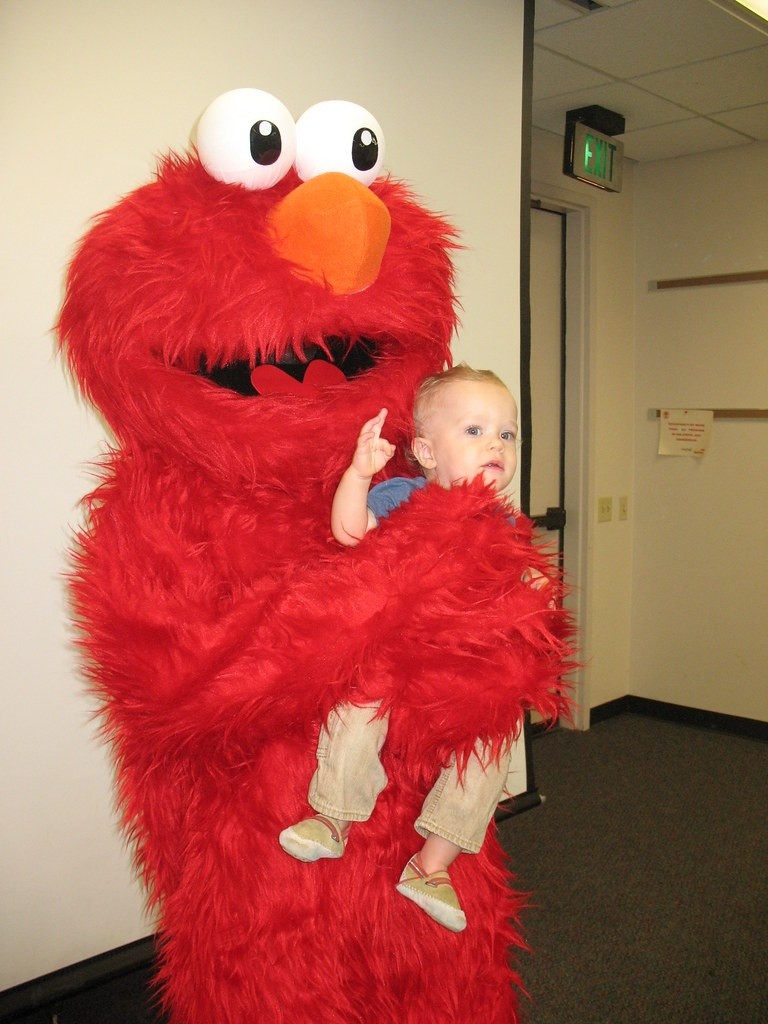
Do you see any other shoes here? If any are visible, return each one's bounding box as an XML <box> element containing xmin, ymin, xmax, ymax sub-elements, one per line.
<box><xmin>278</xmin><ymin>814</ymin><xmax>353</xmax><ymax>863</ymax></box>
<box><xmin>394</xmin><ymin>852</ymin><xmax>467</xmax><ymax>932</ymax></box>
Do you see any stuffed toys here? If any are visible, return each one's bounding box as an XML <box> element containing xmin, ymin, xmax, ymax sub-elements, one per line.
<box><xmin>62</xmin><ymin>86</ymin><xmax>563</xmax><ymax>1024</ymax></box>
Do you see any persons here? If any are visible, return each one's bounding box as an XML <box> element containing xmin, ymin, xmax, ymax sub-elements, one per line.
<box><xmin>281</xmin><ymin>364</ymin><xmax>550</xmax><ymax>931</ymax></box>
